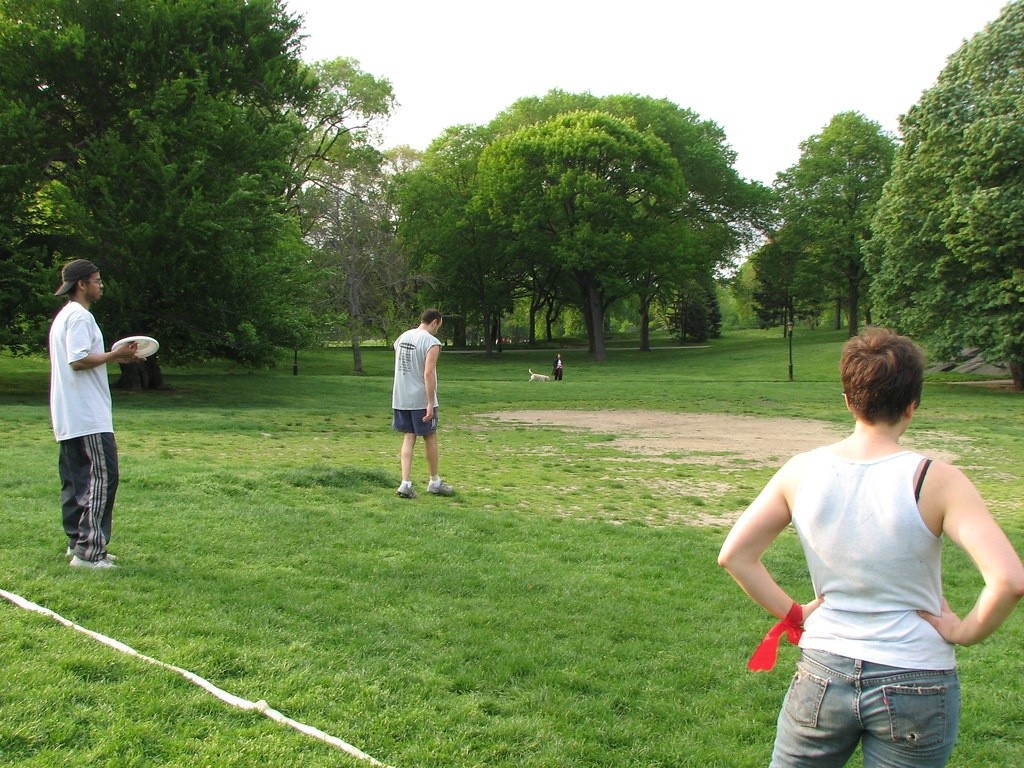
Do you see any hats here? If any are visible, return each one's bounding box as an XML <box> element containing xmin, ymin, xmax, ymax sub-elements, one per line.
<box><xmin>54</xmin><ymin>259</ymin><xmax>99</xmax><ymax>297</ymax></box>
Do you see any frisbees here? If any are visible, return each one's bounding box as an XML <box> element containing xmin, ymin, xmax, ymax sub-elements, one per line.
<box><xmin>110</xmin><ymin>335</ymin><xmax>161</xmax><ymax>359</ymax></box>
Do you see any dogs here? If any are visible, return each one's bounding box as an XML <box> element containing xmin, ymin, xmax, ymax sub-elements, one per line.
<box><xmin>528</xmin><ymin>369</ymin><xmax>550</xmax><ymax>382</ymax></box>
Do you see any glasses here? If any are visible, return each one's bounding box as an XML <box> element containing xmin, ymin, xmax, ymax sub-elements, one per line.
<box><xmin>85</xmin><ymin>277</ymin><xmax>103</xmax><ymax>284</ymax></box>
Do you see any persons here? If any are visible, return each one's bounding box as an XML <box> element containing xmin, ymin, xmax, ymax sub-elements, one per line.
<box><xmin>718</xmin><ymin>328</ymin><xmax>1024</xmax><ymax>768</ymax></box>
<box><xmin>552</xmin><ymin>354</ymin><xmax>562</xmax><ymax>381</ymax></box>
<box><xmin>392</xmin><ymin>309</ymin><xmax>453</xmax><ymax>498</ymax></box>
<box><xmin>48</xmin><ymin>259</ymin><xmax>146</xmax><ymax>569</ymax></box>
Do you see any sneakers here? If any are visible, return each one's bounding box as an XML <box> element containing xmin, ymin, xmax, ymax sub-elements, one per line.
<box><xmin>427</xmin><ymin>480</ymin><xmax>454</xmax><ymax>495</ymax></box>
<box><xmin>395</xmin><ymin>484</ymin><xmax>417</xmax><ymax>498</ymax></box>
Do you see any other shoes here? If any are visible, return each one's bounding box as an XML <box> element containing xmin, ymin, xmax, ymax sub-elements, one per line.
<box><xmin>66</xmin><ymin>546</ymin><xmax>119</xmax><ymax>560</ymax></box>
<box><xmin>70</xmin><ymin>555</ymin><xmax>118</xmax><ymax>569</ymax></box>
